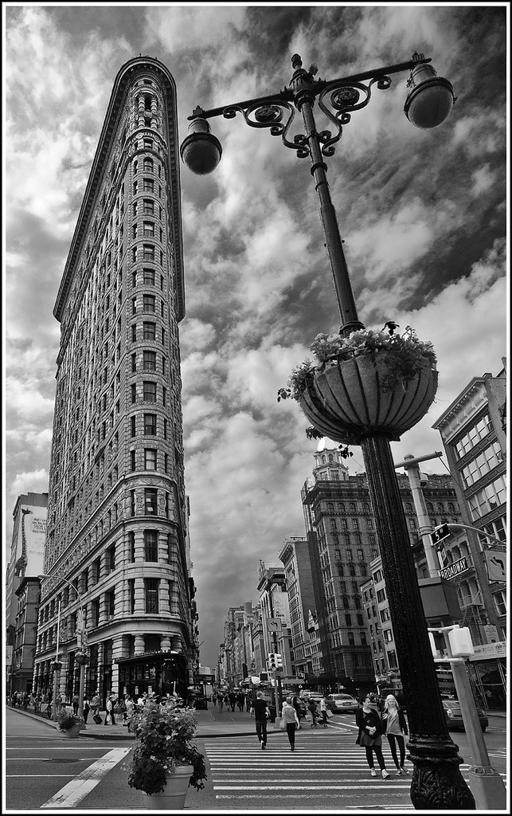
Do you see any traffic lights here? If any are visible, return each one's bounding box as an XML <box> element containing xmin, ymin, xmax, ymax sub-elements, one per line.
<box><xmin>276</xmin><ymin>654</ymin><xmax>282</xmax><ymax>669</ymax></box>
<box><xmin>268</xmin><ymin>653</ymin><xmax>278</xmax><ymax>670</ymax></box>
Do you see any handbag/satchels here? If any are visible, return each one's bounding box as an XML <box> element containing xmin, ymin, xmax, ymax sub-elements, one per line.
<box><xmin>325</xmin><ymin>709</ymin><xmax>334</xmax><ymax>718</ymax></box>
<box><xmin>85</xmin><ymin>703</ymin><xmax>89</xmax><ymax>709</ymax></box>
<box><xmin>362</xmin><ymin>732</ymin><xmax>377</xmax><ymax>747</ymax></box>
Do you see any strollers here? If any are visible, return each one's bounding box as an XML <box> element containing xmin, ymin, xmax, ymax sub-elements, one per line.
<box><xmin>310</xmin><ymin>709</ymin><xmax>328</xmax><ymax>729</ymax></box>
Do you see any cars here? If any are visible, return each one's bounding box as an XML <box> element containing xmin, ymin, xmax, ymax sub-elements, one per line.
<box><xmin>213</xmin><ymin>685</ymin><xmax>359</xmax><ymax>719</ymax></box>
<box><xmin>439</xmin><ymin>694</ymin><xmax>488</xmax><ymax>734</ymax></box>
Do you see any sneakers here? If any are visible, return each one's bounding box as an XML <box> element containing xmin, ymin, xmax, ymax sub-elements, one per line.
<box><xmin>380</xmin><ymin>768</ymin><xmax>392</xmax><ymax>779</ymax></box>
<box><xmin>395</xmin><ymin>770</ymin><xmax>403</xmax><ymax>778</ymax></box>
<box><xmin>260</xmin><ymin>740</ymin><xmax>265</xmax><ymax>749</ymax></box>
<box><xmin>402</xmin><ymin>765</ymin><xmax>411</xmax><ymax>777</ymax></box>
<box><xmin>368</xmin><ymin>768</ymin><xmax>377</xmax><ymax>778</ymax></box>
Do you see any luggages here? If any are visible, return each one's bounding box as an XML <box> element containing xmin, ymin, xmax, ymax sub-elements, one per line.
<box><xmin>88</xmin><ymin>709</ymin><xmax>103</xmax><ymax>725</ymax></box>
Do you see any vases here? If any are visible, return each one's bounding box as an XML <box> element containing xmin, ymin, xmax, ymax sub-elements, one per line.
<box><xmin>298</xmin><ymin>355</ymin><xmax>437</xmax><ymax>443</ymax></box>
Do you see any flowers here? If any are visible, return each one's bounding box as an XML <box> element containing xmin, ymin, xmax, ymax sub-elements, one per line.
<box><xmin>276</xmin><ymin>318</ymin><xmax>437</xmax><ymax>403</ymax></box>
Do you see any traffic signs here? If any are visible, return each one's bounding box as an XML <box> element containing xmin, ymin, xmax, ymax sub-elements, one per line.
<box><xmin>437</xmin><ymin>555</ymin><xmax>470</xmax><ymax>582</ymax></box>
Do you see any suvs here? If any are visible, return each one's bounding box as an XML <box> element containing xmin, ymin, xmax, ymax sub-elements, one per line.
<box><xmin>376</xmin><ymin>688</ymin><xmax>408</xmax><ymax>714</ymax></box>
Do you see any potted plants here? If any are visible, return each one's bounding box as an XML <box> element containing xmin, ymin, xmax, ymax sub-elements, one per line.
<box><xmin>123</xmin><ymin>690</ymin><xmax>208</xmax><ymax>811</ymax></box>
<box><xmin>56</xmin><ymin>710</ymin><xmax>85</xmax><ymax>739</ymax></box>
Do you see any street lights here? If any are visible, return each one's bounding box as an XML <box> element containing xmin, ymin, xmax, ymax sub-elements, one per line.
<box><xmin>257</xmin><ymin>573</ymin><xmax>285</xmax><ymax>717</ymax></box>
<box><xmin>38</xmin><ymin>575</ymin><xmax>87</xmax><ymax>730</ymax></box>
<box><xmin>179</xmin><ymin>53</ymin><xmax>476</xmax><ymax>810</ymax></box>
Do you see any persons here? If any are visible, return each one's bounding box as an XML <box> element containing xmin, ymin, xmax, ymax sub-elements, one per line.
<box><xmin>10</xmin><ymin>690</ymin><xmax>147</xmax><ymax>726</ymax></box>
<box><xmin>211</xmin><ymin>688</ymin><xmax>328</xmax><ymax>751</ymax></box>
<box><xmin>381</xmin><ymin>695</ymin><xmax>410</xmax><ymax>776</ymax></box>
<box><xmin>355</xmin><ymin>697</ymin><xmax>391</xmax><ymax>779</ymax></box>
